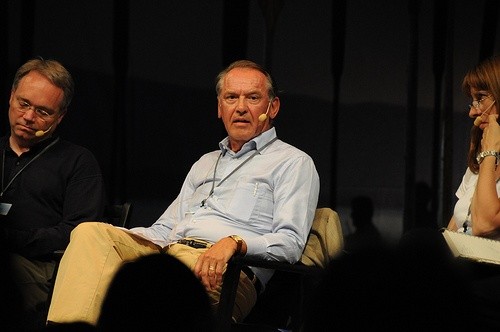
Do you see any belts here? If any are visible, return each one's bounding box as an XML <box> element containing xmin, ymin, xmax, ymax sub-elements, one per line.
<box><xmin>178</xmin><ymin>240</ymin><xmax>263</xmax><ymax>302</ymax></box>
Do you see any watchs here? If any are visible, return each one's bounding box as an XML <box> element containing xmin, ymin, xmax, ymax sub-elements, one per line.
<box><xmin>228</xmin><ymin>234</ymin><xmax>242</xmax><ymax>255</ymax></box>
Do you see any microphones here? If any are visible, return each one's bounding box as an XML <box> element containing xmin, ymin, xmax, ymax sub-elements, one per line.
<box><xmin>35</xmin><ymin>120</ymin><xmax>58</xmax><ymax>137</ymax></box>
<box><xmin>473</xmin><ymin>100</ymin><xmax>496</xmax><ymax>127</ymax></box>
<box><xmin>258</xmin><ymin>98</ymin><xmax>273</xmax><ymax>121</ymax></box>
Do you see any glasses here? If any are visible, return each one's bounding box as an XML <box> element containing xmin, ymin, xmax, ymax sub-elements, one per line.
<box><xmin>468</xmin><ymin>93</ymin><xmax>493</xmax><ymax>110</ymax></box>
<box><xmin>13</xmin><ymin>90</ymin><xmax>60</xmax><ymax>121</ymax></box>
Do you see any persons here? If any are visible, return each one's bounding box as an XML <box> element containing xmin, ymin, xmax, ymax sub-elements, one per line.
<box><xmin>95</xmin><ymin>253</ymin><xmax>214</xmax><ymax>332</ymax></box>
<box><xmin>0</xmin><ymin>57</ymin><xmax>104</xmax><ymax>326</ymax></box>
<box><xmin>385</xmin><ymin>52</ymin><xmax>500</xmax><ymax>331</ymax></box>
<box><xmin>46</xmin><ymin>60</ymin><xmax>322</xmax><ymax>332</ymax></box>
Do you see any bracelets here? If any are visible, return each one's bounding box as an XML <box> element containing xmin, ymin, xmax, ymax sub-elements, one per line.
<box><xmin>476</xmin><ymin>150</ymin><xmax>498</xmax><ymax>165</ymax></box>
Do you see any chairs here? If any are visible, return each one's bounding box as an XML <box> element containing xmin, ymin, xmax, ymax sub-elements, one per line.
<box><xmin>216</xmin><ymin>208</ymin><xmax>343</xmax><ymax>332</ymax></box>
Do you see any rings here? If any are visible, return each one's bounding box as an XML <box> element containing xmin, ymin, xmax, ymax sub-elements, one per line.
<box><xmin>209</xmin><ymin>267</ymin><xmax>215</xmax><ymax>271</ymax></box>
